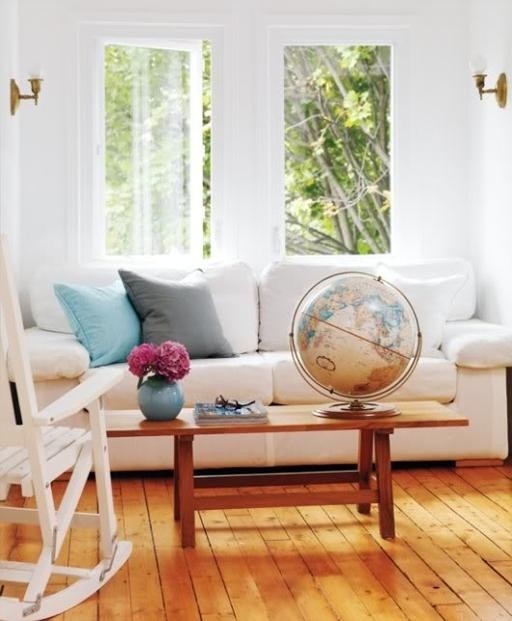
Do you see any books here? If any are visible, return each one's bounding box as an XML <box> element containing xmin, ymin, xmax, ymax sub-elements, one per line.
<box><xmin>193</xmin><ymin>402</ymin><xmax>270</xmax><ymax>425</ymax></box>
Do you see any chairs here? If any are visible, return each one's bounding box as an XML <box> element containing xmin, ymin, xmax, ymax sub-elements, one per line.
<box><xmin>1</xmin><ymin>233</ymin><xmax>138</xmax><ymax>621</ymax></box>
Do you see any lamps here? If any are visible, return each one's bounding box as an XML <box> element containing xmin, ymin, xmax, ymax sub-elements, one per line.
<box><xmin>11</xmin><ymin>51</ymin><xmax>43</xmax><ymax>113</ymax></box>
<box><xmin>468</xmin><ymin>51</ymin><xmax>508</xmax><ymax>107</ymax></box>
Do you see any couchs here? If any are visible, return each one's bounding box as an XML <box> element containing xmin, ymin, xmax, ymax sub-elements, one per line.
<box><xmin>14</xmin><ymin>251</ymin><xmax>510</xmax><ymax>475</ymax></box>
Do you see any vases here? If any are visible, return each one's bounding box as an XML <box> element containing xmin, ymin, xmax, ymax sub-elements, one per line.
<box><xmin>137</xmin><ymin>378</ymin><xmax>185</xmax><ymax>421</ymax></box>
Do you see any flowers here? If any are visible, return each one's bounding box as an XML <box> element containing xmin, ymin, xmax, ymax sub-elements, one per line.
<box><xmin>127</xmin><ymin>341</ymin><xmax>192</xmax><ymax>387</ymax></box>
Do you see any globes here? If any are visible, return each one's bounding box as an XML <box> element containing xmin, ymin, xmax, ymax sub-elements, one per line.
<box><xmin>288</xmin><ymin>271</ymin><xmax>422</xmax><ymax>420</ymax></box>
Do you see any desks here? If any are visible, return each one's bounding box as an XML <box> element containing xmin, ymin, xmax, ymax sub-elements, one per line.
<box><xmin>98</xmin><ymin>399</ymin><xmax>470</xmax><ymax>548</ymax></box>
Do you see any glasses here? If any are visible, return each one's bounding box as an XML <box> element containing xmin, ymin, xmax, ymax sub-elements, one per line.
<box><xmin>215</xmin><ymin>394</ymin><xmax>255</xmax><ymax>410</ymax></box>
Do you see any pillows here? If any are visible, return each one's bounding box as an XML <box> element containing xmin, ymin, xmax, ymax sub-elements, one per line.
<box><xmin>51</xmin><ymin>277</ymin><xmax>141</xmax><ymax>368</ymax></box>
<box><xmin>373</xmin><ymin>264</ymin><xmax>457</xmax><ymax>360</ymax></box>
<box><xmin>117</xmin><ymin>263</ymin><xmax>236</xmax><ymax>359</ymax></box>
